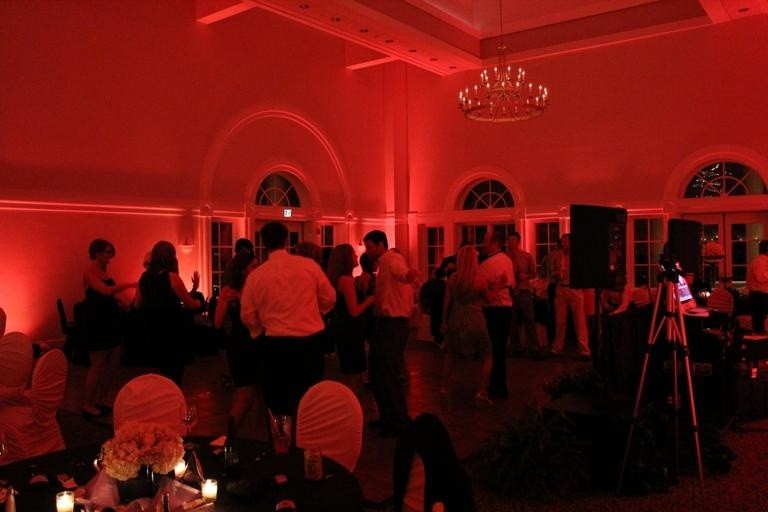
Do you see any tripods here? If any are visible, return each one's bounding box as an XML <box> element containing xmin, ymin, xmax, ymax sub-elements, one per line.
<box><xmin>627</xmin><ymin>273</ymin><xmax>709</xmax><ymax>492</ymax></box>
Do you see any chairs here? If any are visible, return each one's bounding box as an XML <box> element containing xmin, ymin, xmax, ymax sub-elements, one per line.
<box><xmin>2</xmin><ymin>331</ymin><xmax>33</xmax><ymax>406</ymax></box>
<box><xmin>1</xmin><ymin>348</ymin><xmax>67</xmax><ymax>464</ymax></box>
<box><xmin>297</xmin><ymin>380</ymin><xmax>362</xmax><ymax>471</ymax></box>
<box><xmin>113</xmin><ymin>375</ymin><xmax>188</xmax><ymax>436</ymax></box>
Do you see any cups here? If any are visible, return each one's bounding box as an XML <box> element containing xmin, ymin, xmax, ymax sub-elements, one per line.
<box><xmin>304</xmin><ymin>449</ymin><xmax>322</xmax><ymax>480</ymax></box>
<box><xmin>175</xmin><ymin>458</ymin><xmax>184</xmax><ymax>476</ymax></box>
<box><xmin>201</xmin><ymin>478</ymin><xmax>217</xmax><ymax>500</ymax></box>
<box><xmin>272</xmin><ymin>415</ymin><xmax>292</xmax><ymax>455</ymax></box>
<box><xmin>56</xmin><ymin>490</ymin><xmax>75</xmax><ymax>512</ymax></box>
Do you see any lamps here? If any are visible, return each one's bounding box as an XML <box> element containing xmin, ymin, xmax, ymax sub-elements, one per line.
<box><xmin>458</xmin><ymin>0</ymin><xmax>550</xmax><ymax>124</ymax></box>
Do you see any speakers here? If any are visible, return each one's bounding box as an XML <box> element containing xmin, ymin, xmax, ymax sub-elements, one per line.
<box><xmin>569</xmin><ymin>204</ymin><xmax>627</xmax><ymax>291</ymax></box>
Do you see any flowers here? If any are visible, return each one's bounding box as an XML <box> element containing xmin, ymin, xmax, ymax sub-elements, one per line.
<box><xmin>101</xmin><ymin>423</ymin><xmax>184</xmax><ymax>480</ymax></box>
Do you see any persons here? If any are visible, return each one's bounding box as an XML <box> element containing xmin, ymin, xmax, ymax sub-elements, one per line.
<box><xmin>241</xmin><ymin>222</ymin><xmax>336</xmax><ymax>443</ymax></box>
<box><xmin>357</xmin><ymin>252</ymin><xmax>379</xmax><ymax>388</ymax></box>
<box><xmin>216</xmin><ymin>252</ymin><xmax>257</xmax><ymax>440</ymax></box>
<box><xmin>430</xmin><ymin>240</ymin><xmax>507</xmax><ymax>406</ymax></box>
<box><xmin>479</xmin><ymin>230</ymin><xmax>516</xmax><ymax>400</ymax></box>
<box><xmin>539</xmin><ymin>235</ymin><xmax>590</xmax><ymax>356</ymax></box>
<box><xmin>71</xmin><ymin>237</ymin><xmax>138</xmax><ymax>417</ymax></box>
<box><xmin>598</xmin><ymin>239</ymin><xmax>768</xmax><ymax>339</ymax></box>
<box><xmin>326</xmin><ymin>243</ymin><xmax>377</xmax><ymax>392</ymax></box>
<box><xmin>505</xmin><ymin>231</ymin><xmax>546</xmax><ymax>360</ymax></box>
<box><xmin>235</xmin><ymin>238</ymin><xmax>261</xmax><ymax>268</ymax></box>
<box><xmin>132</xmin><ymin>241</ymin><xmax>205</xmax><ymax>390</ymax></box>
<box><xmin>364</xmin><ymin>229</ymin><xmax>420</xmax><ymax>432</ymax></box>
<box><xmin>295</xmin><ymin>242</ymin><xmax>321</xmax><ymax>263</ymax></box>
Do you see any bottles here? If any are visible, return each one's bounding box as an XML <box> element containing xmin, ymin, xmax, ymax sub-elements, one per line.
<box><xmin>5</xmin><ymin>485</ymin><xmax>17</xmax><ymax>511</ymax></box>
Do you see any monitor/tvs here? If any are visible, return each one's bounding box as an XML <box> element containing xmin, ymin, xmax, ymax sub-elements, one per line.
<box><xmin>667</xmin><ymin>218</ymin><xmax>702</xmax><ymax>273</ymax></box>
<box><xmin>669</xmin><ymin>275</ymin><xmax>696</xmax><ymax>310</ymax></box>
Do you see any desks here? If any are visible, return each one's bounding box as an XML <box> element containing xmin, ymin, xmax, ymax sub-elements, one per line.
<box><xmin>4</xmin><ymin>437</ymin><xmax>365</xmax><ymax>510</ymax></box>
